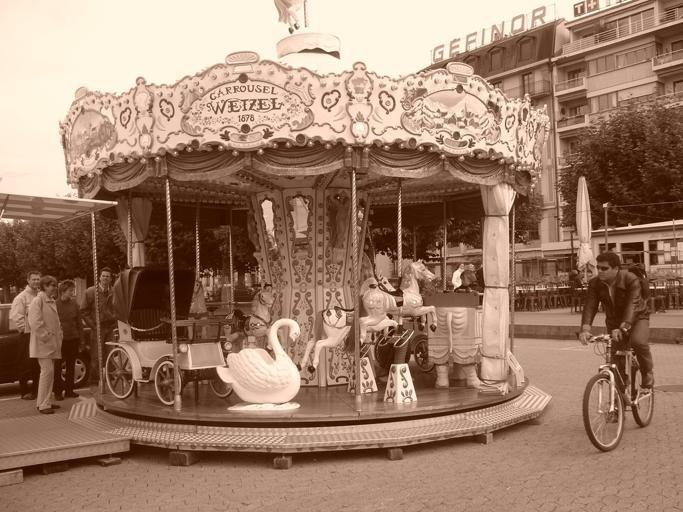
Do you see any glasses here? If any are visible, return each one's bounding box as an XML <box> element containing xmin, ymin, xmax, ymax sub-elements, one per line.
<box><xmin>596</xmin><ymin>264</ymin><xmax>611</xmax><ymax>271</ymax></box>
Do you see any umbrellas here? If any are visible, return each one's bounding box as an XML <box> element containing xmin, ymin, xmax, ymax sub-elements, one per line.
<box><xmin>576</xmin><ymin>174</ymin><xmax>596</xmax><ymax>283</ymax></box>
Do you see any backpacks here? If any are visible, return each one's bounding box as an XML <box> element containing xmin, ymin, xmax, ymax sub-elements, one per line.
<box><xmin>628</xmin><ymin>262</ymin><xmax>651</xmax><ymax>301</ymax></box>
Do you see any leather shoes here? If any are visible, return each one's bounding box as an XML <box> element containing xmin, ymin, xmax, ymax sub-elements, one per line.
<box><xmin>641</xmin><ymin>369</ymin><xmax>655</xmax><ymax>389</ymax></box>
<box><xmin>608</xmin><ymin>409</ymin><xmax>625</xmax><ymax>422</ymax></box>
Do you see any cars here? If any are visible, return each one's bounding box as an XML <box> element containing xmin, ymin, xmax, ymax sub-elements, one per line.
<box><xmin>0</xmin><ymin>304</ymin><xmax>119</xmax><ymax>392</ymax></box>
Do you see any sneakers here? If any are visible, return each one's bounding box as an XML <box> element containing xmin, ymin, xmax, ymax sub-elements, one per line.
<box><xmin>37</xmin><ymin>403</ymin><xmax>61</xmax><ymax>409</ymax></box>
<box><xmin>20</xmin><ymin>393</ymin><xmax>36</xmax><ymax>400</ymax></box>
<box><xmin>54</xmin><ymin>392</ymin><xmax>65</xmax><ymax>401</ymax></box>
<box><xmin>64</xmin><ymin>392</ymin><xmax>80</xmax><ymax>398</ymax></box>
<box><xmin>39</xmin><ymin>406</ymin><xmax>55</xmax><ymax>415</ymax></box>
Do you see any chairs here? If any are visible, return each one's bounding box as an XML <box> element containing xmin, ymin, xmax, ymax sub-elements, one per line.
<box><xmin>511</xmin><ymin>270</ymin><xmax>682</xmax><ymax>314</ymax></box>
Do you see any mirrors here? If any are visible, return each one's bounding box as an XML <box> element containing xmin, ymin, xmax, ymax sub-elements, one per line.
<box><xmin>326</xmin><ymin>190</ymin><xmax>349</xmax><ymax>248</ymax></box>
<box><xmin>285</xmin><ymin>193</ymin><xmax>314</xmax><ymax>258</ymax></box>
<box><xmin>258</xmin><ymin>195</ymin><xmax>279</xmax><ymax>262</ymax></box>
<box><xmin>356</xmin><ymin>200</ymin><xmax>368</xmax><ymax>244</ymax></box>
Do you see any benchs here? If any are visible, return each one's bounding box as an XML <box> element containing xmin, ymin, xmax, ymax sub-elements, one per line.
<box><xmin>127</xmin><ymin>306</ymin><xmax>172</xmax><ymax>339</ymax></box>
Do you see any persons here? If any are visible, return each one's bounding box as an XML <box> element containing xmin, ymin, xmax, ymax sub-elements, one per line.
<box><xmin>579</xmin><ymin>251</ymin><xmax>654</xmax><ymax>424</ymax></box>
<box><xmin>8</xmin><ymin>271</ymin><xmax>43</xmax><ymax>400</ymax></box>
<box><xmin>27</xmin><ymin>275</ymin><xmax>64</xmax><ymax>413</ymax></box>
<box><xmin>628</xmin><ymin>256</ymin><xmax>648</xmax><ymax>280</ymax></box>
<box><xmin>569</xmin><ymin>267</ymin><xmax>583</xmax><ymax>288</ymax></box>
<box><xmin>451</xmin><ymin>263</ymin><xmax>485</xmax><ymax>306</ymax></box>
<box><xmin>54</xmin><ymin>280</ymin><xmax>80</xmax><ymax>400</ymax></box>
<box><xmin>80</xmin><ymin>267</ymin><xmax>116</xmax><ymax>383</ymax></box>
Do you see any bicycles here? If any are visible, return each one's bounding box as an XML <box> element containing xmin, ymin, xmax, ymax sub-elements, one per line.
<box><xmin>574</xmin><ymin>325</ymin><xmax>654</xmax><ymax>453</ymax></box>
